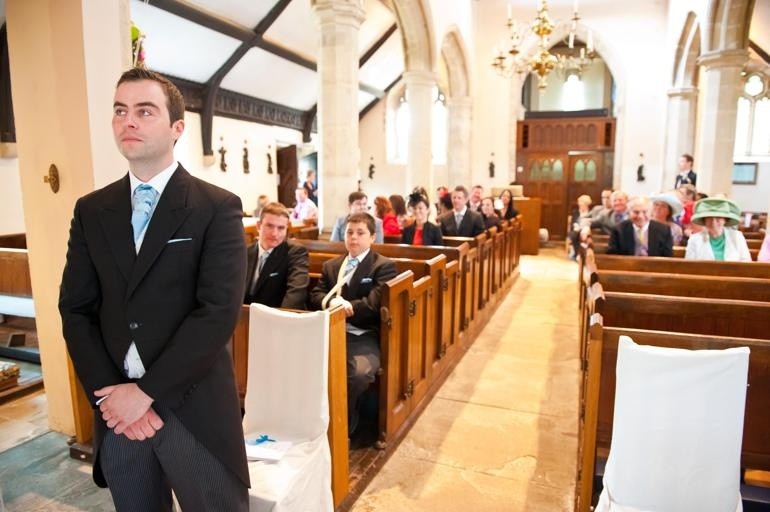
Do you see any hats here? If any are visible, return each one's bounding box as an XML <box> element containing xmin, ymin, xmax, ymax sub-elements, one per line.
<box><xmin>691</xmin><ymin>193</ymin><xmax>743</xmax><ymax>229</ymax></box>
<box><xmin>647</xmin><ymin>190</ymin><xmax>684</xmax><ymax>216</ymax></box>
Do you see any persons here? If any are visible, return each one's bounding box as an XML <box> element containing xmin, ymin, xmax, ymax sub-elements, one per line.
<box><xmin>566</xmin><ymin>154</ymin><xmax>769</xmax><ymax>263</ymax></box>
<box><xmin>57</xmin><ymin>68</ymin><xmax>252</xmax><ymax>512</ymax></box>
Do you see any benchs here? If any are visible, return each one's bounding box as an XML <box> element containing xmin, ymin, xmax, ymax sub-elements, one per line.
<box><xmin>566</xmin><ymin>214</ymin><xmax>770</xmax><ymax>512</ymax></box>
<box><xmin>0</xmin><ymin>233</ymin><xmax>35</xmax><ymax>320</ymax></box>
<box><xmin>238</xmin><ymin>212</ymin><xmax>524</xmax><ymax>510</ymax></box>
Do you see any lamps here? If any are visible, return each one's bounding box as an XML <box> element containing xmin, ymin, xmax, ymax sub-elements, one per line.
<box><xmin>488</xmin><ymin>0</ymin><xmax>597</xmax><ymax>88</ymax></box>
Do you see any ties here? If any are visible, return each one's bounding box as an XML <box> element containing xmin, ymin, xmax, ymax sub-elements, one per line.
<box><xmin>636</xmin><ymin>228</ymin><xmax>644</xmax><ymax>245</ymax></box>
<box><xmin>343</xmin><ymin>259</ymin><xmax>358</xmax><ymax>277</ymax></box>
<box><xmin>129</xmin><ymin>183</ymin><xmax>156</xmax><ymax>241</ymax></box>
<box><xmin>260</xmin><ymin>252</ymin><xmax>270</xmax><ymax>270</ymax></box>
